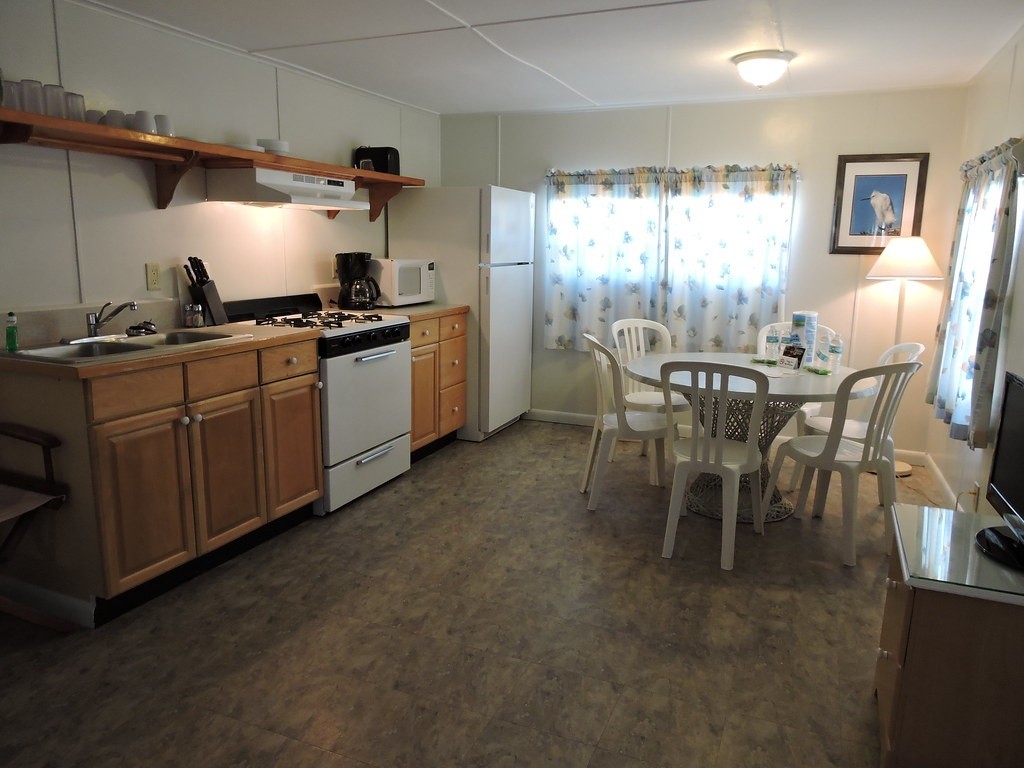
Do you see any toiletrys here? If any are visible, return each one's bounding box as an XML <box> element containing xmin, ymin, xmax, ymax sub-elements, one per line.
<box><xmin>5</xmin><ymin>312</ymin><xmax>19</xmax><ymax>354</ymax></box>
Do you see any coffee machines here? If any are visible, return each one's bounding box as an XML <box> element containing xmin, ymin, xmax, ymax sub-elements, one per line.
<box><xmin>334</xmin><ymin>252</ymin><xmax>372</xmax><ymax>310</ymax></box>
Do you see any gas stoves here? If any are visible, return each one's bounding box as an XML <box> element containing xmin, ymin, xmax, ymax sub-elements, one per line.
<box><xmin>221</xmin><ymin>293</ymin><xmax>411</xmax><ymax>360</ymax></box>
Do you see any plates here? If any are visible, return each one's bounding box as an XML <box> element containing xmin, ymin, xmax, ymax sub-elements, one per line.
<box><xmin>224</xmin><ymin>138</ymin><xmax>291</xmax><ymax>157</ymax></box>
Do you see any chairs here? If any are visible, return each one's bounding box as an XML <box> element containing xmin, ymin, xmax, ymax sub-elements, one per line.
<box><xmin>581</xmin><ymin>333</ymin><xmax>689</xmax><ymax>517</ymax></box>
<box><xmin>659</xmin><ymin>360</ymin><xmax>769</xmax><ymax>571</ymax></box>
<box><xmin>804</xmin><ymin>343</ymin><xmax>925</xmax><ymax>520</ymax></box>
<box><xmin>756</xmin><ymin>323</ymin><xmax>836</xmax><ymax>461</ymax></box>
<box><xmin>762</xmin><ymin>361</ymin><xmax>925</xmax><ymax>567</ymax></box>
<box><xmin>607</xmin><ymin>319</ymin><xmax>705</xmax><ymax>462</ymax></box>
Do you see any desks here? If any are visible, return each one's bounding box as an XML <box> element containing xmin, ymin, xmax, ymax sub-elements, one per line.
<box><xmin>625</xmin><ymin>352</ymin><xmax>879</xmax><ymax>521</ymax></box>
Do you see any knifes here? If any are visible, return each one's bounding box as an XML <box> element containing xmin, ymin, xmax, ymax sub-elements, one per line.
<box><xmin>182</xmin><ymin>256</ymin><xmax>209</xmax><ymax>286</ymax></box>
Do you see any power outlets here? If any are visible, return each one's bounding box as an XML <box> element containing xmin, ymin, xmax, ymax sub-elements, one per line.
<box><xmin>145</xmin><ymin>262</ymin><xmax>161</xmax><ymax>290</ymax></box>
<box><xmin>972</xmin><ymin>481</ymin><xmax>980</xmax><ymax>512</ymax></box>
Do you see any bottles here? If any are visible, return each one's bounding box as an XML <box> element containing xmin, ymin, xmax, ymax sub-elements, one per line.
<box><xmin>184</xmin><ymin>304</ymin><xmax>204</xmax><ymax>328</ymax></box>
<box><xmin>6</xmin><ymin>312</ymin><xmax>19</xmax><ymax>351</ymax></box>
<box><xmin>765</xmin><ymin>324</ymin><xmax>843</xmax><ymax>375</ymax></box>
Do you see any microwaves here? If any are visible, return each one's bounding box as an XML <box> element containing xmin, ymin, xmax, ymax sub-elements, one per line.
<box><xmin>367</xmin><ymin>258</ymin><xmax>436</xmax><ymax>306</ymax></box>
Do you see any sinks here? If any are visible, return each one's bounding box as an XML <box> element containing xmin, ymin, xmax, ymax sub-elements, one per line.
<box><xmin>107</xmin><ymin>330</ymin><xmax>254</xmax><ymax>348</ymax></box>
<box><xmin>14</xmin><ymin>339</ymin><xmax>171</xmax><ymax>363</ymax></box>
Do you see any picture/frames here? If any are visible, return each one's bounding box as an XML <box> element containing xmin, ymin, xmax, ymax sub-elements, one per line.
<box><xmin>829</xmin><ymin>152</ymin><xmax>929</xmax><ymax>255</ymax></box>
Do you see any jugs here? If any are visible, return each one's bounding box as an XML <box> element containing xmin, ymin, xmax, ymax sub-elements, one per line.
<box><xmin>349</xmin><ymin>274</ymin><xmax>381</xmax><ymax>303</ymax></box>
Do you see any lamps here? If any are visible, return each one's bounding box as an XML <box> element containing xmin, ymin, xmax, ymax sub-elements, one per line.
<box><xmin>864</xmin><ymin>237</ymin><xmax>944</xmax><ymax>476</ymax></box>
<box><xmin>732</xmin><ymin>49</ymin><xmax>795</xmax><ymax>89</ymax></box>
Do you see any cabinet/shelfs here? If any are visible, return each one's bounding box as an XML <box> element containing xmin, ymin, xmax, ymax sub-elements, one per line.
<box><xmin>875</xmin><ymin>502</ymin><xmax>1024</xmax><ymax>768</ymax></box>
<box><xmin>410</xmin><ymin>314</ymin><xmax>467</xmax><ymax>452</ymax></box>
<box><xmin>85</xmin><ymin>338</ymin><xmax>323</xmax><ymax>601</ymax></box>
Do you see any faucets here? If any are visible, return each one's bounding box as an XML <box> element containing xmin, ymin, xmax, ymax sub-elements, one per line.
<box><xmin>86</xmin><ymin>301</ymin><xmax>138</xmax><ymax>337</ymax></box>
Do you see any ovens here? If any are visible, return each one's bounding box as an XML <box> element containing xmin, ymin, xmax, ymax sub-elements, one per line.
<box><xmin>317</xmin><ymin>340</ymin><xmax>411</xmax><ymax>512</ymax></box>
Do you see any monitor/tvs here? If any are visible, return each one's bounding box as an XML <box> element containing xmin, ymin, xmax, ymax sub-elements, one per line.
<box><xmin>974</xmin><ymin>370</ymin><xmax>1024</xmax><ymax>573</ymax></box>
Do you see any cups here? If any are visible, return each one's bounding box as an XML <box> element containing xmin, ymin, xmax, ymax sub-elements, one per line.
<box><xmin>0</xmin><ymin>79</ymin><xmax>175</xmax><ymax>139</ymax></box>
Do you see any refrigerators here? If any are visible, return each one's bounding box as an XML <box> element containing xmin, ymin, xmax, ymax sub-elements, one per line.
<box><xmin>386</xmin><ymin>183</ymin><xmax>538</xmax><ymax>442</ymax></box>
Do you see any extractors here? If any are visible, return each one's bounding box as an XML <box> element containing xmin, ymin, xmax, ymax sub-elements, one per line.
<box><xmin>206</xmin><ymin>168</ymin><xmax>371</xmax><ymax>212</ymax></box>
